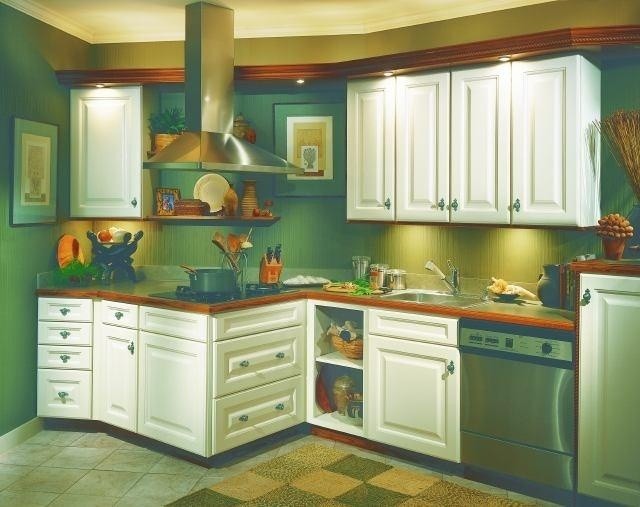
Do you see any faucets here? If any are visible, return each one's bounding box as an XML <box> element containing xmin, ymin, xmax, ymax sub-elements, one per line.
<box><xmin>424</xmin><ymin>260</ymin><xmax>459</xmax><ymax>296</ymax></box>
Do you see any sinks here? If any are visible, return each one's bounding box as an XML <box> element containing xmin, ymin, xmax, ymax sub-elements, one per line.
<box><xmin>379</xmin><ymin>292</ymin><xmax>487</xmax><ymax>308</ymax></box>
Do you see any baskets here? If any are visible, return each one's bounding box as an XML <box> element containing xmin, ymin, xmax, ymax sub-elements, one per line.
<box><xmin>333</xmin><ymin>336</ymin><xmax>362</xmax><ymax>360</ymax></box>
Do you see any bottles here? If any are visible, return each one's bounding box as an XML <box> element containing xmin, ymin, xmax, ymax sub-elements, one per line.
<box><xmin>369</xmin><ymin>268</ymin><xmax>384</xmax><ymax>289</ymax></box>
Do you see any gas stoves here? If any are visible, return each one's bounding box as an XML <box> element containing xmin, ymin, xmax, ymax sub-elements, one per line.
<box><xmin>147</xmin><ymin>285</ymin><xmax>302</xmax><ymax>304</ymax></box>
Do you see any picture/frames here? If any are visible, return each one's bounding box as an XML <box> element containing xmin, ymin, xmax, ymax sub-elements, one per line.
<box><xmin>156</xmin><ymin>188</ymin><xmax>180</xmax><ymax>216</ymax></box>
<box><xmin>11</xmin><ymin>117</ymin><xmax>61</xmax><ymax>226</ymax></box>
<box><xmin>273</xmin><ymin>103</ymin><xmax>347</xmax><ymax>198</ymax></box>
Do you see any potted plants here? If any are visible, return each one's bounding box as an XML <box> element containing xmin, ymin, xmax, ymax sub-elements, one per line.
<box><xmin>147</xmin><ymin>105</ymin><xmax>186</xmax><ymax>157</ymax></box>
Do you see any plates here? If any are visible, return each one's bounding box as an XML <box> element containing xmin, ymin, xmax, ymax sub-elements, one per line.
<box><xmin>495</xmin><ymin>292</ymin><xmax>521</xmax><ymax>300</ymax></box>
<box><xmin>193</xmin><ymin>171</ymin><xmax>230</xmax><ymax>215</ymax></box>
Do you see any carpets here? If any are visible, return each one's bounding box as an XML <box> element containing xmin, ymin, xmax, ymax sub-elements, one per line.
<box><xmin>161</xmin><ymin>443</ymin><xmax>544</xmax><ymax>507</ymax></box>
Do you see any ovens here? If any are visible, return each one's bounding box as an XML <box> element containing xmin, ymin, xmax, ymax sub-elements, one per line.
<box><xmin>460</xmin><ymin>324</ymin><xmax>577</xmax><ymax>495</ymax></box>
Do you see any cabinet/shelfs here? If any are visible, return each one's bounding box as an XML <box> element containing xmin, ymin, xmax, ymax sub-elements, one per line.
<box><xmin>70</xmin><ymin>86</ymin><xmax>160</xmax><ymax>220</ymax></box>
<box><xmin>92</xmin><ymin>300</ymin><xmax>212</xmax><ymax>469</ymax></box>
<box><xmin>37</xmin><ymin>297</ymin><xmax>92</xmax><ymax>432</ymax></box>
<box><xmin>306</xmin><ymin>299</ymin><xmax>460</xmax><ymax>477</ymax></box>
<box><xmin>347</xmin><ymin>77</ymin><xmax>396</xmax><ymax>224</ymax></box>
<box><xmin>394</xmin><ymin>62</ymin><xmax>510</xmax><ymax>228</ymax></box>
<box><xmin>210</xmin><ymin>298</ymin><xmax>307</xmax><ymax>468</ymax></box>
<box><xmin>509</xmin><ymin>54</ymin><xmax>602</xmax><ymax>231</ymax></box>
<box><xmin>569</xmin><ymin>263</ymin><xmax>640</xmax><ymax>507</ymax></box>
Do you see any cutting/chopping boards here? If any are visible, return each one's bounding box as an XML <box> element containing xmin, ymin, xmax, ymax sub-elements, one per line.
<box><xmin>322</xmin><ymin>282</ymin><xmax>355</xmax><ymax>292</ymax></box>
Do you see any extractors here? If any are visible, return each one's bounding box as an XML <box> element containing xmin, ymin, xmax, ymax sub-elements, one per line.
<box><xmin>141</xmin><ymin>2</ymin><xmax>304</xmax><ymax>176</ymax></box>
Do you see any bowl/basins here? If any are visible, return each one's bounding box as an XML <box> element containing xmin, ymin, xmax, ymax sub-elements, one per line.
<box><xmin>174</xmin><ymin>197</ymin><xmax>209</xmax><ymax>215</ymax></box>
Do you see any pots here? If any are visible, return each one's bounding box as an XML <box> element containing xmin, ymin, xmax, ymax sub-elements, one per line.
<box><xmin>183</xmin><ymin>268</ymin><xmax>244</xmax><ymax>297</ymax></box>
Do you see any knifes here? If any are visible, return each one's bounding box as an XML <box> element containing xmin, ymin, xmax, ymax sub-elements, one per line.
<box><xmin>266</xmin><ymin>242</ymin><xmax>282</xmax><ymax>263</ymax></box>
